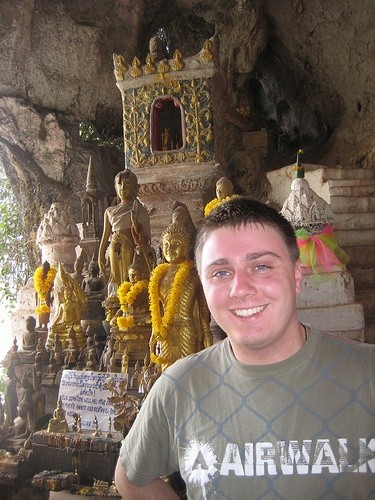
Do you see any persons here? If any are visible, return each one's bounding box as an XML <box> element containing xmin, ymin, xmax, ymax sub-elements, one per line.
<box><xmin>52</xmin><ymin>289</ymin><xmax>85</xmax><ymax>337</ymax></box>
<box><xmin>168</xmin><ymin>200</ymin><xmax>199</xmax><ymax>233</ymax></box>
<box><xmin>206</xmin><ymin>176</ymin><xmax>240</xmax><ymax>217</ymax></box>
<box><xmin>146</xmin><ymin>224</ymin><xmax>215</xmax><ymax>375</ymax></box>
<box><xmin>110</xmin><ymin>196</ymin><xmax>375</xmax><ymax>500</ymax></box>
<box><xmin>112</xmin><ymin>251</ymin><xmax>153</xmax><ymax>329</ymax></box>
<box><xmin>0</xmin><ymin>317</ymin><xmax>162</xmax><ymax>460</ymax></box>
<box><xmin>97</xmin><ymin>166</ymin><xmax>151</xmax><ymax>284</ymax></box>
